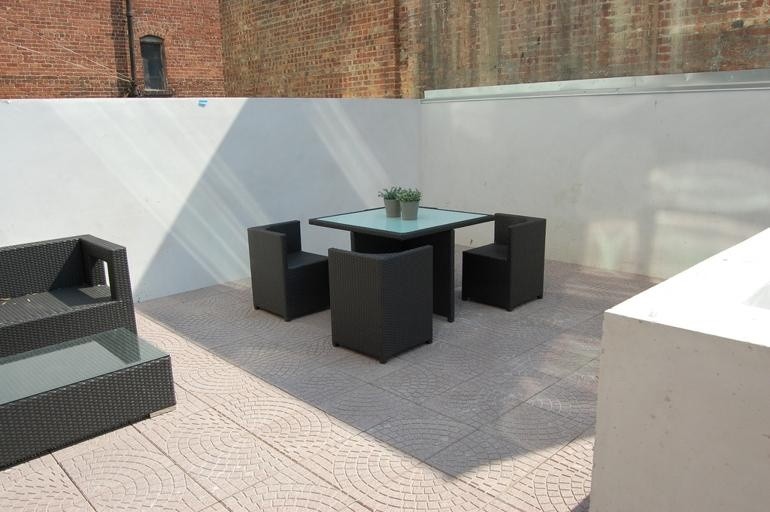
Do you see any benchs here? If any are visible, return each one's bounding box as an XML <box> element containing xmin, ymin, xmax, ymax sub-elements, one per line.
<box><xmin>0</xmin><ymin>234</ymin><xmax>140</xmax><ymax>357</ymax></box>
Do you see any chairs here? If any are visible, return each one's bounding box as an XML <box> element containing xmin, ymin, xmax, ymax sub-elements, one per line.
<box><xmin>326</xmin><ymin>246</ymin><xmax>436</xmax><ymax>364</ymax></box>
<box><xmin>248</xmin><ymin>218</ymin><xmax>328</xmax><ymax>322</ymax></box>
<box><xmin>461</xmin><ymin>212</ymin><xmax>546</xmax><ymax>312</ymax></box>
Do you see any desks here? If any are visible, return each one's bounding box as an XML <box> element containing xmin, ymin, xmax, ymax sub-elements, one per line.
<box><xmin>307</xmin><ymin>207</ymin><xmax>495</xmax><ymax>323</ymax></box>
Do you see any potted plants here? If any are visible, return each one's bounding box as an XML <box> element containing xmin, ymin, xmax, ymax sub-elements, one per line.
<box><xmin>376</xmin><ymin>183</ymin><xmax>422</xmax><ymax>221</ymax></box>
